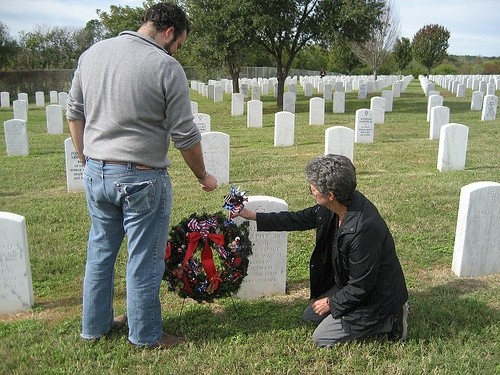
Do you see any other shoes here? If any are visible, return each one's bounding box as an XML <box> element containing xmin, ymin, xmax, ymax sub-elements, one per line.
<box><xmin>107</xmin><ymin>314</ymin><xmax>127</xmax><ymax>336</ymax></box>
<box><xmin>146</xmin><ymin>334</ymin><xmax>186</xmax><ymax>349</ymax></box>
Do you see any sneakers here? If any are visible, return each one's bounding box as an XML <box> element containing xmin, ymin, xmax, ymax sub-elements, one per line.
<box><xmin>387</xmin><ymin>302</ymin><xmax>408</xmax><ymax>342</ymax></box>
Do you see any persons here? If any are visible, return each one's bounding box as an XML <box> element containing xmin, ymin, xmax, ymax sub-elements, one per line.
<box><xmin>320</xmin><ymin>68</ymin><xmax>326</xmax><ymax>78</ymax></box>
<box><xmin>230</xmin><ymin>154</ymin><xmax>410</xmax><ymax>346</ymax></box>
<box><xmin>65</xmin><ymin>3</ymin><xmax>217</xmax><ymax>348</ymax></box>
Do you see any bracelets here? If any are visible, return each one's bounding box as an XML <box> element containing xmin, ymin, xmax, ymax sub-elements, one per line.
<box><xmin>197</xmin><ymin>171</ymin><xmax>209</xmax><ymax>180</ymax></box>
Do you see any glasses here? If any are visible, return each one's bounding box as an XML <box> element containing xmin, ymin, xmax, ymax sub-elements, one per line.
<box><xmin>309</xmin><ymin>186</ymin><xmax>324</xmax><ymax>196</ymax></box>
